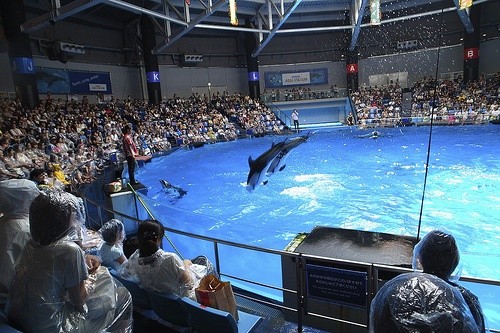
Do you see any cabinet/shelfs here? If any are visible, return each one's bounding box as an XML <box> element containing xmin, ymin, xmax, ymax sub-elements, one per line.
<box><xmin>102</xmin><ymin>180</ymin><xmax>148</xmax><ymax>236</ymax></box>
<box><xmin>281</xmin><ymin>232</ymin><xmax>414</xmax><ymax>333</ymax></box>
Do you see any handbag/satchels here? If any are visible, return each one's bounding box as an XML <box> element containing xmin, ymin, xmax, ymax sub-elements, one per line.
<box><xmin>195</xmin><ymin>275</ymin><xmax>240</xmax><ymax>322</ymax></box>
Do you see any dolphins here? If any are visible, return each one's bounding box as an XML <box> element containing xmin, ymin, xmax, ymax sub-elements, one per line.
<box><xmin>246</xmin><ymin>128</ymin><xmax>315</xmax><ymax>193</ymax></box>
<box><xmin>158</xmin><ymin>178</ymin><xmax>186</xmax><ymax>198</ymax></box>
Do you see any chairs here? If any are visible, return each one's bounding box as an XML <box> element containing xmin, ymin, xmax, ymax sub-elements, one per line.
<box><xmin>0</xmin><ymin>248</ymin><xmax>264</xmax><ymax>333</ymax></box>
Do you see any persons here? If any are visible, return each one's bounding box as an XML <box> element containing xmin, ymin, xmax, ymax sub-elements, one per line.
<box><xmin>121</xmin><ymin>124</ymin><xmax>141</xmax><ymax>185</ymax></box>
<box><xmin>0</xmin><ymin>72</ymin><xmax>500</xmax><ymax>333</ymax></box>
